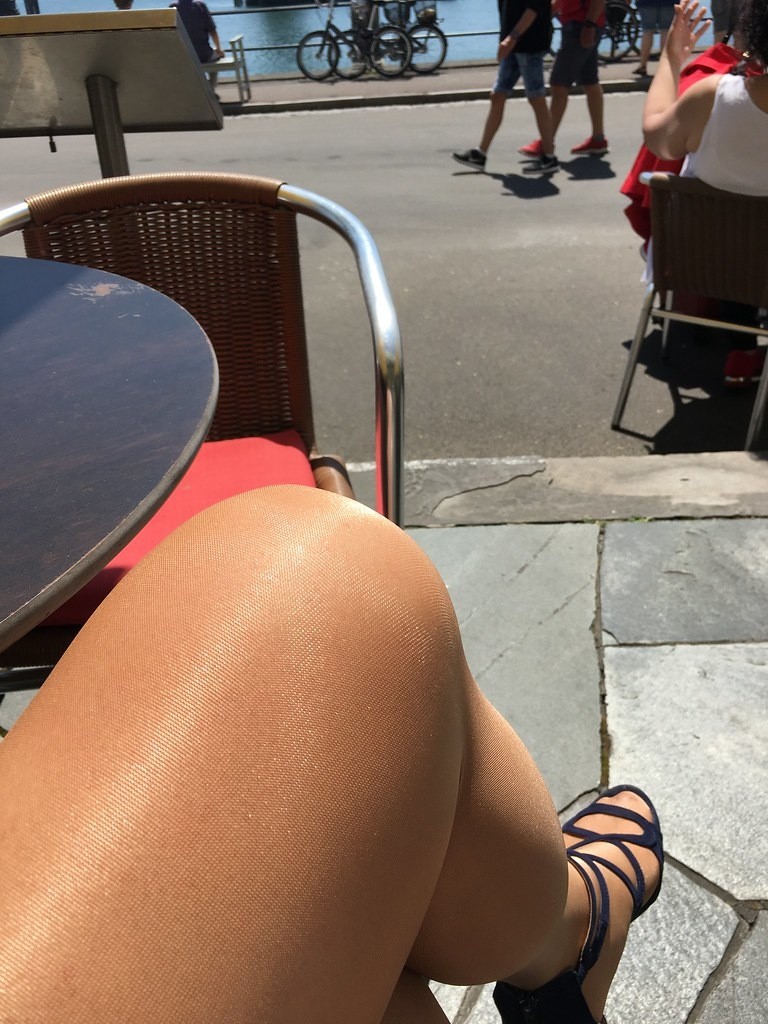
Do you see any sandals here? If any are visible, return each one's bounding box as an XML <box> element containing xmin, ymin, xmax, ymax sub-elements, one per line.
<box><xmin>492</xmin><ymin>784</ymin><xmax>664</xmax><ymax>1024</ymax></box>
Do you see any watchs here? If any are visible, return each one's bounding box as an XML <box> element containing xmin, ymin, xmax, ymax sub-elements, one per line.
<box><xmin>509</xmin><ymin>30</ymin><xmax>520</xmax><ymax>39</ymax></box>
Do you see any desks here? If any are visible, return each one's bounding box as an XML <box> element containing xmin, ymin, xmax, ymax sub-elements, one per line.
<box><xmin>1</xmin><ymin>255</ymin><xmax>220</xmax><ymax>653</ymax></box>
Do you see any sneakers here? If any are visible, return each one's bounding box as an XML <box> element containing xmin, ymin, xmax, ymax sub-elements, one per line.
<box><xmin>518</xmin><ymin>140</ymin><xmax>543</xmax><ymax>157</ymax></box>
<box><xmin>570</xmin><ymin>136</ymin><xmax>608</xmax><ymax>154</ymax></box>
<box><xmin>523</xmin><ymin>154</ymin><xmax>559</xmax><ymax>174</ymax></box>
<box><xmin>450</xmin><ymin>149</ymin><xmax>486</xmax><ymax>172</ymax></box>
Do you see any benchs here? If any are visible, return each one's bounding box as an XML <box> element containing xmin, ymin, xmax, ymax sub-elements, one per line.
<box><xmin>202</xmin><ymin>35</ymin><xmax>251</xmax><ymax>105</ymax></box>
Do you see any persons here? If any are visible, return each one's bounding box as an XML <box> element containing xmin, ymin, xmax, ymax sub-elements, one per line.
<box><xmin>643</xmin><ymin>0</ymin><xmax>768</xmax><ymax>386</ymax></box>
<box><xmin>0</xmin><ymin>485</ymin><xmax>663</xmax><ymax>1024</ymax></box>
<box><xmin>632</xmin><ymin>0</ymin><xmax>680</xmax><ymax>74</ymax></box>
<box><xmin>449</xmin><ymin>0</ymin><xmax>561</xmax><ymax>174</ymax></box>
<box><xmin>711</xmin><ymin>0</ymin><xmax>751</xmax><ymax>50</ymax></box>
<box><xmin>170</xmin><ymin>0</ymin><xmax>224</xmax><ymax>100</ymax></box>
<box><xmin>348</xmin><ymin>1</ymin><xmax>381</xmax><ymax>75</ymax></box>
<box><xmin>113</xmin><ymin>0</ymin><xmax>134</xmax><ymax>10</ymax></box>
<box><xmin>518</xmin><ymin>0</ymin><xmax>609</xmax><ymax>161</ymax></box>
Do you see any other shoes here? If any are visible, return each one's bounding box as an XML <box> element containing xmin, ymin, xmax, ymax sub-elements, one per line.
<box><xmin>667</xmin><ymin>341</ymin><xmax>706</xmax><ymax>386</ymax></box>
<box><xmin>723</xmin><ymin>349</ymin><xmax>766</xmax><ymax>384</ymax></box>
<box><xmin>633</xmin><ymin>66</ymin><xmax>646</xmax><ymax>74</ymax></box>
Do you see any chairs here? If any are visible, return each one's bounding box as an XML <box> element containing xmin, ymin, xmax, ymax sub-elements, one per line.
<box><xmin>0</xmin><ymin>173</ymin><xmax>404</xmax><ymax>711</ymax></box>
<box><xmin>610</xmin><ymin>172</ymin><xmax>768</xmax><ymax>453</ymax></box>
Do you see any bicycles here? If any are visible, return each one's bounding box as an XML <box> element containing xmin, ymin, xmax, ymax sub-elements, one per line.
<box><xmin>288</xmin><ymin>1</ymin><xmax>448</xmax><ymax>84</ymax></box>
<box><xmin>546</xmin><ymin>1</ymin><xmax>665</xmax><ymax>62</ymax></box>
